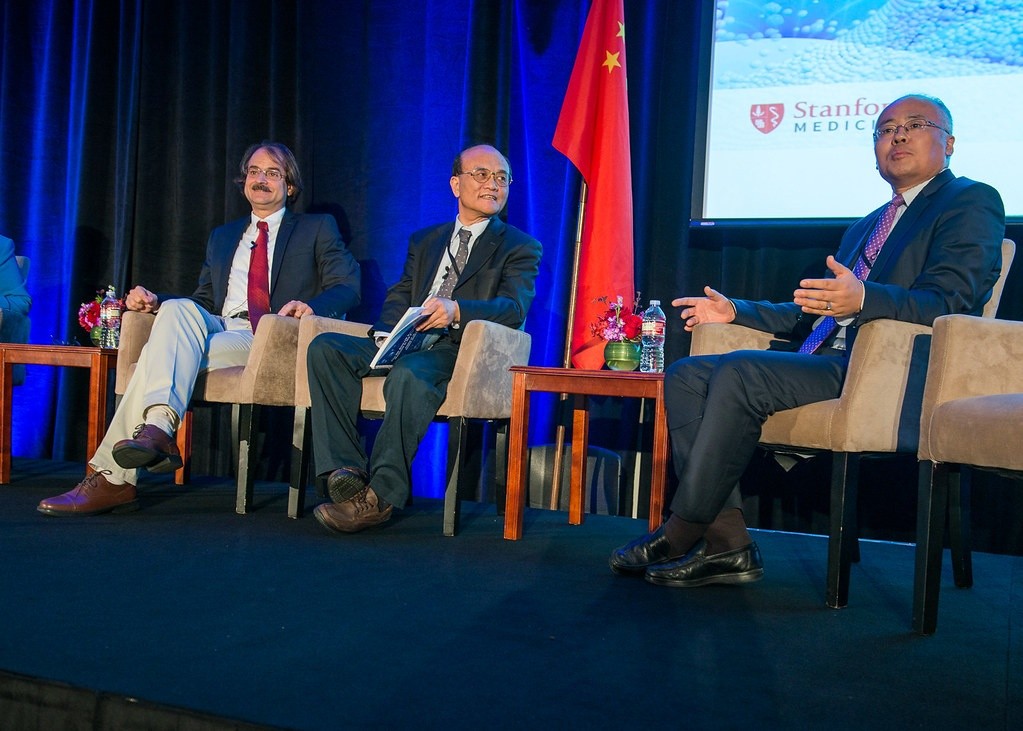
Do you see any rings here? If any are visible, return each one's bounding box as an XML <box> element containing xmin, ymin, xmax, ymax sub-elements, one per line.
<box><xmin>825</xmin><ymin>300</ymin><xmax>831</xmax><ymax>311</ymax></box>
<box><xmin>296</xmin><ymin>309</ymin><xmax>303</xmax><ymax>314</ymax></box>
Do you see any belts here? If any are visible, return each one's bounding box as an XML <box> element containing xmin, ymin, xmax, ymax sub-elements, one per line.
<box><xmin>230</xmin><ymin>310</ymin><xmax>249</xmax><ymax>321</ymax></box>
<box><xmin>830</xmin><ymin>336</ymin><xmax>846</xmax><ymax>351</ymax></box>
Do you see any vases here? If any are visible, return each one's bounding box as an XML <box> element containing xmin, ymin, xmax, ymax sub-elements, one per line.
<box><xmin>90</xmin><ymin>326</ymin><xmax>119</xmax><ymax>348</ymax></box>
<box><xmin>603</xmin><ymin>340</ymin><xmax>640</xmax><ymax>372</ymax></box>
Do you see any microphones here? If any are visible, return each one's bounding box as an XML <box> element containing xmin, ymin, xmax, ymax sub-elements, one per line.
<box><xmin>870</xmin><ymin>248</ymin><xmax>880</xmax><ymax>260</ymax></box>
<box><xmin>250</xmin><ymin>239</ymin><xmax>259</xmax><ymax>251</ymax></box>
<box><xmin>442</xmin><ymin>266</ymin><xmax>450</xmax><ymax>279</ymax></box>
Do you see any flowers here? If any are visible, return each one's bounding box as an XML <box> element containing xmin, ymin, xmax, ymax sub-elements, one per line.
<box><xmin>78</xmin><ymin>284</ymin><xmax>128</xmax><ymax>332</ymax></box>
<box><xmin>589</xmin><ymin>291</ymin><xmax>643</xmax><ymax>343</ymax></box>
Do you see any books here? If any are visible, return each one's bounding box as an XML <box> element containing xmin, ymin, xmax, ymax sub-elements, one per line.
<box><xmin>370</xmin><ymin>307</ymin><xmax>443</xmax><ymax>369</ymax></box>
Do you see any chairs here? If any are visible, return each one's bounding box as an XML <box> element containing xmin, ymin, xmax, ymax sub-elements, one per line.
<box><xmin>286</xmin><ymin>315</ymin><xmax>532</xmax><ymax>536</ymax></box>
<box><xmin>113</xmin><ymin>309</ymin><xmax>347</xmax><ymax>515</ymax></box>
<box><xmin>690</xmin><ymin>238</ymin><xmax>1015</xmax><ymax>610</ymax></box>
<box><xmin>914</xmin><ymin>313</ymin><xmax>1023</xmax><ymax>636</ymax></box>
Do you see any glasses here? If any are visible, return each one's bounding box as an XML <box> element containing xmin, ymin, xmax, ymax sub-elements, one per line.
<box><xmin>246</xmin><ymin>168</ymin><xmax>287</xmax><ymax>182</ymax></box>
<box><xmin>873</xmin><ymin>118</ymin><xmax>951</xmax><ymax>140</ymax></box>
<box><xmin>460</xmin><ymin>168</ymin><xmax>513</xmax><ymax>187</ymax></box>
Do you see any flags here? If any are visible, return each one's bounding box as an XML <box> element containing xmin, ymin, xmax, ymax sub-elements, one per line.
<box><xmin>553</xmin><ymin>0</ymin><xmax>634</xmax><ymax>370</ymax></box>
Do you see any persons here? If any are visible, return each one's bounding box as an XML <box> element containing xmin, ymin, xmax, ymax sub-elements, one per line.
<box><xmin>38</xmin><ymin>141</ymin><xmax>360</xmax><ymax>518</ymax></box>
<box><xmin>608</xmin><ymin>92</ymin><xmax>1004</xmax><ymax>587</ymax></box>
<box><xmin>305</xmin><ymin>145</ymin><xmax>541</xmax><ymax>535</ymax></box>
<box><xmin>0</xmin><ymin>235</ymin><xmax>33</xmax><ymax>315</ymax></box>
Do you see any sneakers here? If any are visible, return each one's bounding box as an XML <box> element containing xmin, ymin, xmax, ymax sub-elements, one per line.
<box><xmin>313</xmin><ymin>484</ymin><xmax>394</xmax><ymax>537</ymax></box>
<box><xmin>327</xmin><ymin>465</ymin><xmax>370</xmax><ymax>502</ymax></box>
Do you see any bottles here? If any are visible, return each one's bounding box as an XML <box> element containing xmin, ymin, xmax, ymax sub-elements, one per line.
<box><xmin>100</xmin><ymin>290</ymin><xmax>121</xmax><ymax>349</ymax></box>
<box><xmin>640</xmin><ymin>299</ymin><xmax>664</xmax><ymax>372</ymax></box>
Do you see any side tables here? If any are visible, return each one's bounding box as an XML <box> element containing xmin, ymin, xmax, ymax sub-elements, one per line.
<box><xmin>503</xmin><ymin>366</ymin><xmax>668</xmax><ymax>540</ymax></box>
<box><xmin>0</xmin><ymin>342</ymin><xmax>193</xmax><ymax>485</ymax></box>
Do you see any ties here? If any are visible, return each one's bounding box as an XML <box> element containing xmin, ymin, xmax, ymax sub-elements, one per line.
<box><xmin>247</xmin><ymin>221</ymin><xmax>272</xmax><ymax>336</ymax></box>
<box><xmin>797</xmin><ymin>194</ymin><xmax>905</xmax><ymax>355</ymax></box>
<box><xmin>435</xmin><ymin>228</ymin><xmax>473</xmax><ymax>300</ymax></box>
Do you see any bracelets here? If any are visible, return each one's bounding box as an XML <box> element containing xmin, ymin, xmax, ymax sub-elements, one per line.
<box><xmin>450</xmin><ymin>301</ymin><xmax>460</xmax><ymax>330</ymax></box>
<box><xmin>847</xmin><ymin>281</ymin><xmax>862</xmax><ymax>318</ymax></box>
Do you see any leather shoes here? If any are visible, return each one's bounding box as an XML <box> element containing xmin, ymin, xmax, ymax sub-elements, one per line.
<box><xmin>641</xmin><ymin>537</ymin><xmax>765</xmax><ymax>588</ymax></box>
<box><xmin>37</xmin><ymin>470</ymin><xmax>141</xmax><ymax>517</ymax></box>
<box><xmin>112</xmin><ymin>423</ymin><xmax>184</xmax><ymax>474</ymax></box>
<box><xmin>608</xmin><ymin>520</ymin><xmax>687</xmax><ymax>577</ymax></box>
